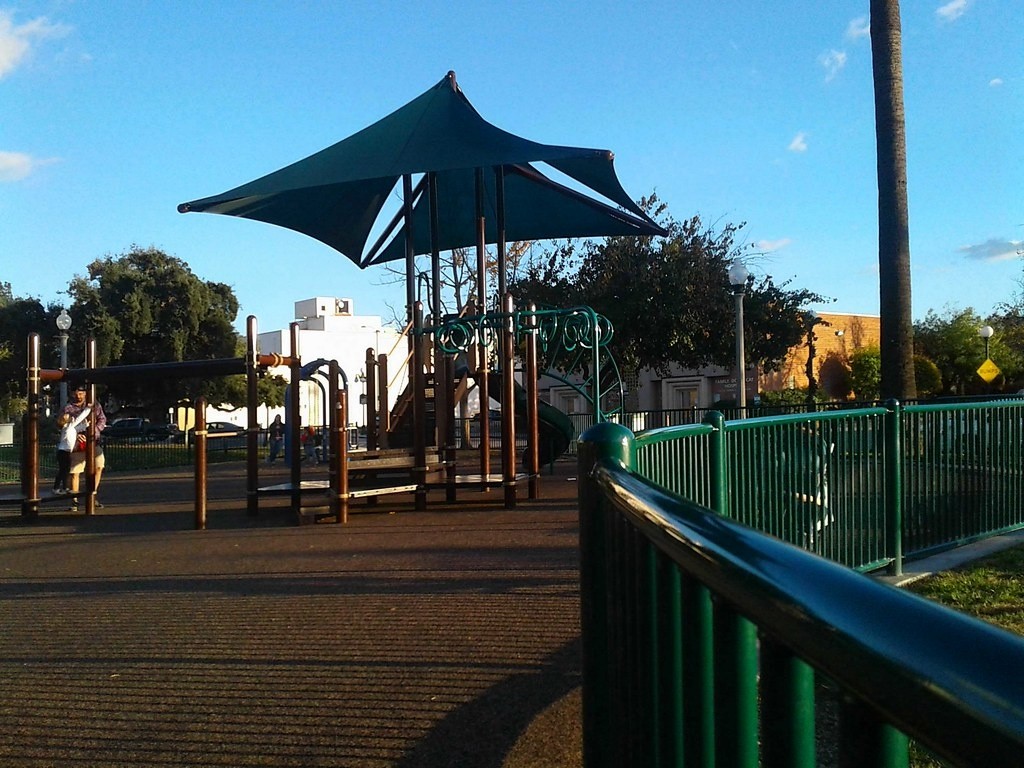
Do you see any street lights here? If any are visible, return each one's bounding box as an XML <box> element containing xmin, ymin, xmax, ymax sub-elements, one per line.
<box><xmin>354</xmin><ymin>368</ymin><xmax>367</xmax><ymax>428</ymax></box>
<box><xmin>729</xmin><ymin>257</ymin><xmax>752</xmax><ymax>420</ymax></box>
<box><xmin>803</xmin><ymin>307</ymin><xmax>818</xmax><ymax>403</ymax></box>
<box><xmin>976</xmin><ymin>326</ymin><xmax>1001</xmax><ymax>394</ymax></box>
<box><xmin>57</xmin><ymin>309</ymin><xmax>73</xmax><ymax>416</ymax></box>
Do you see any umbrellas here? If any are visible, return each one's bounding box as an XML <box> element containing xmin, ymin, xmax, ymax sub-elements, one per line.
<box><xmin>176</xmin><ymin>70</ymin><xmax>670</xmax><ymax>269</ymax></box>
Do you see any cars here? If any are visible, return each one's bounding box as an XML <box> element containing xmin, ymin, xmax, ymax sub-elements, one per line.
<box><xmin>172</xmin><ymin>422</ymin><xmax>244</xmax><ymax>444</ymax></box>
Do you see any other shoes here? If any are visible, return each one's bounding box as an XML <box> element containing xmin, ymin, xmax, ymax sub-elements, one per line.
<box><xmin>95</xmin><ymin>501</ymin><xmax>103</xmax><ymax>510</ymax></box>
<box><xmin>52</xmin><ymin>488</ymin><xmax>69</xmax><ymax>495</ymax></box>
<box><xmin>69</xmin><ymin>502</ymin><xmax>79</xmax><ymax>511</ymax></box>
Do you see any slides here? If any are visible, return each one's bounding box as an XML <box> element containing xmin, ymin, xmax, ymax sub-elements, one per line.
<box><xmin>472</xmin><ymin>373</ymin><xmax>574</xmax><ymax>468</ymax></box>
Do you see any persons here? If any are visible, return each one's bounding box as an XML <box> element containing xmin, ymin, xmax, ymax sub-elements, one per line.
<box><xmin>62</xmin><ymin>383</ymin><xmax>107</xmax><ymax>512</ymax></box>
<box><xmin>266</xmin><ymin>414</ymin><xmax>285</xmax><ymax>468</ymax></box>
<box><xmin>301</xmin><ymin>425</ymin><xmax>319</xmax><ymax>468</ymax></box>
<box><xmin>53</xmin><ymin>402</ymin><xmax>92</xmax><ymax>495</ymax></box>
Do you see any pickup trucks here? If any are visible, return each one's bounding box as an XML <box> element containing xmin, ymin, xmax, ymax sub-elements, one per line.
<box><xmin>100</xmin><ymin>419</ymin><xmax>177</xmax><ymax>443</ymax></box>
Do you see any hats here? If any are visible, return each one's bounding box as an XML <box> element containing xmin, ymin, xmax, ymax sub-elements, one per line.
<box><xmin>71</xmin><ymin>383</ymin><xmax>86</xmax><ymax>391</ymax></box>
